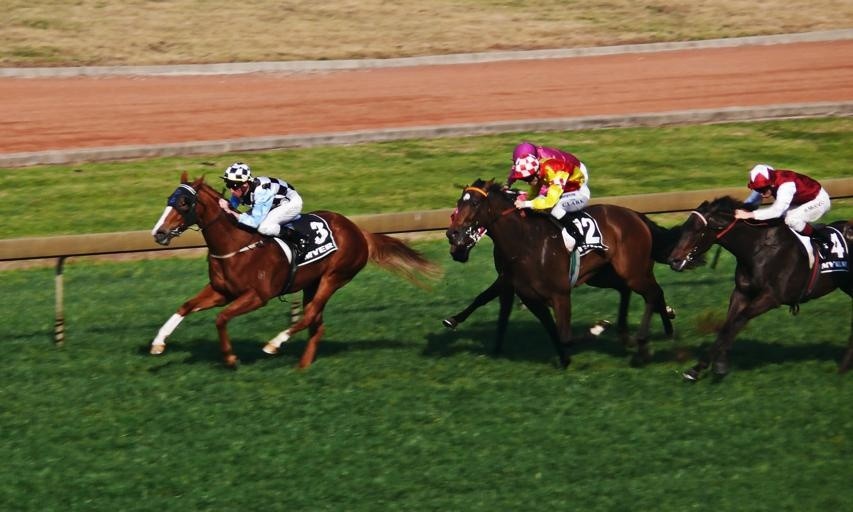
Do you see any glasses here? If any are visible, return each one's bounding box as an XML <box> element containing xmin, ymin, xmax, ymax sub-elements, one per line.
<box><xmin>754</xmin><ymin>185</ymin><xmax>771</xmax><ymax>193</ymax></box>
<box><xmin>225</xmin><ymin>182</ymin><xmax>246</xmax><ymax>191</ymax></box>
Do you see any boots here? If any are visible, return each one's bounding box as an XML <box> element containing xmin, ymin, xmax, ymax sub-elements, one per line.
<box><xmin>558</xmin><ymin>211</ymin><xmax>585</xmax><ymax>252</ymax></box>
<box><xmin>276</xmin><ymin>224</ymin><xmax>310</xmax><ymax>258</ymax></box>
<box><xmin>810</xmin><ymin>228</ymin><xmax>834</xmax><ymax>257</ymax></box>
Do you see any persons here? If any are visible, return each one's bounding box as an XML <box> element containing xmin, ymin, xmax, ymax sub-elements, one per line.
<box><xmin>735</xmin><ymin>164</ymin><xmax>831</xmax><ymax>252</ymax></box>
<box><xmin>502</xmin><ymin>142</ymin><xmax>590</xmax><ymax>247</ymax></box>
<box><xmin>219</xmin><ymin>162</ymin><xmax>310</xmax><ymax>264</ymax></box>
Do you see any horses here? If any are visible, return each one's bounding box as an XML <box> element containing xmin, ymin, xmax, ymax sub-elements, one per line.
<box><xmin>667</xmin><ymin>193</ymin><xmax>853</xmax><ymax>384</ymax></box>
<box><xmin>442</xmin><ymin>176</ymin><xmax>707</xmax><ymax>368</ymax></box>
<box><xmin>149</xmin><ymin>169</ymin><xmax>446</xmax><ymax>373</ymax></box>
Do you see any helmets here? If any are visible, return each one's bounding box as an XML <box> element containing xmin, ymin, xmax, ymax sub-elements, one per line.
<box><xmin>219</xmin><ymin>162</ymin><xmax>251</xmax><ymax>183</ymax></box>
<box><xmin>747</xmin><ymin>164</ymin><xmax>775</xmax><ymax>189</ymax></box>
<box><xmin>509</xmin><ymin>153</ymin><xmax>539</xmax><ymax>180</ymax></box>
<box><xmin>513</xmin><ymin>142</ymin><xmax>537</xmax><ymax>163</ymax></box>
